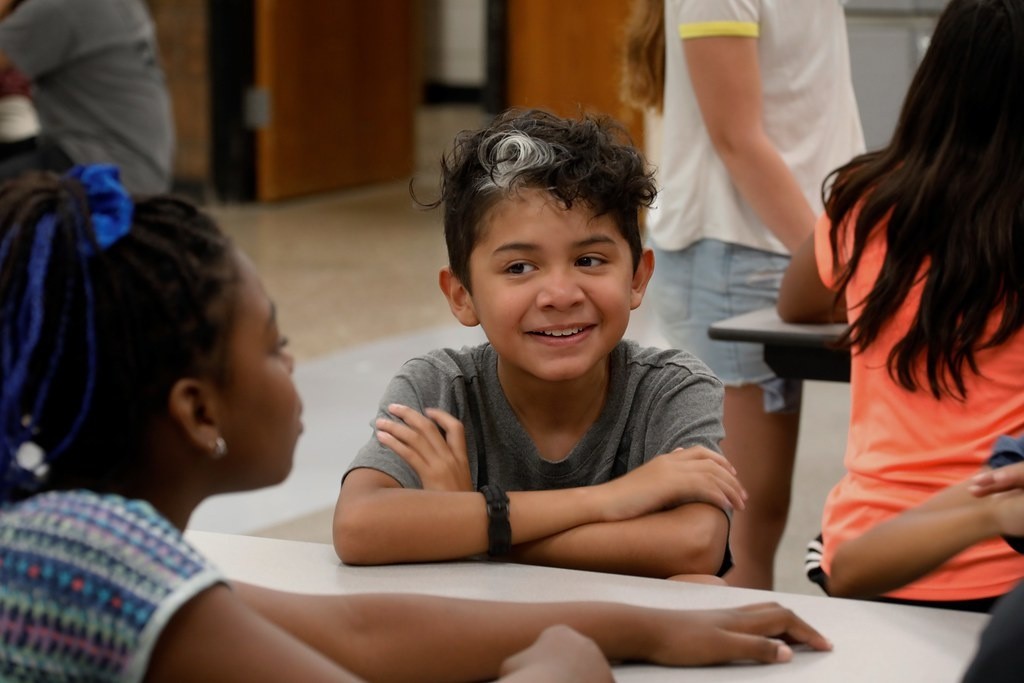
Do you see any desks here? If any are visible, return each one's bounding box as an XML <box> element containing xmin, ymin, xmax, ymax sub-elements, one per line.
<box><xmin>183</xmin><ymin>530</ymin><xmax>991</xmax><ymax>683</ymax></box>
<box><xmin>707</xmin><ymin>305</ymin><xmax>851</xmax><ymax>384</ymax></box>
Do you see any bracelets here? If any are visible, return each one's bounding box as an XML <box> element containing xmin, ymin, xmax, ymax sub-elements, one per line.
<box><xmin>480</xmin><ymin>480</ymin><xmax>517</xmax><ymax>562</ymax></box>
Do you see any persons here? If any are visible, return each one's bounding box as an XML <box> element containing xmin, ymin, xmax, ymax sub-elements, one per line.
<box><xmin>0</xmin><ymin>0</ymin><xmax>179</xmax><ymax>197</ymax></box>
<box><xmin>774</xmin><ymin>1</ymin><xmax>1024</xmax><ymax>613</ymax></box>
<box><xmin>831</xmin><ymin>429</ymin><xmax>1024</xmax><ymax>683</ymax></box>
<box><xmin>330</xmin><ymin>106</ymin><xmax>772</xmax><ymax>593</ymax></box>
<box><xmin>626</xmin><ymin>1</ymin><xmax>869</xmax><ymax>592</ymax></box>
<box><xmin>0</xmin><ymin>169</ymin><xmax>837</xmax><ymax>683</ymax></box>
